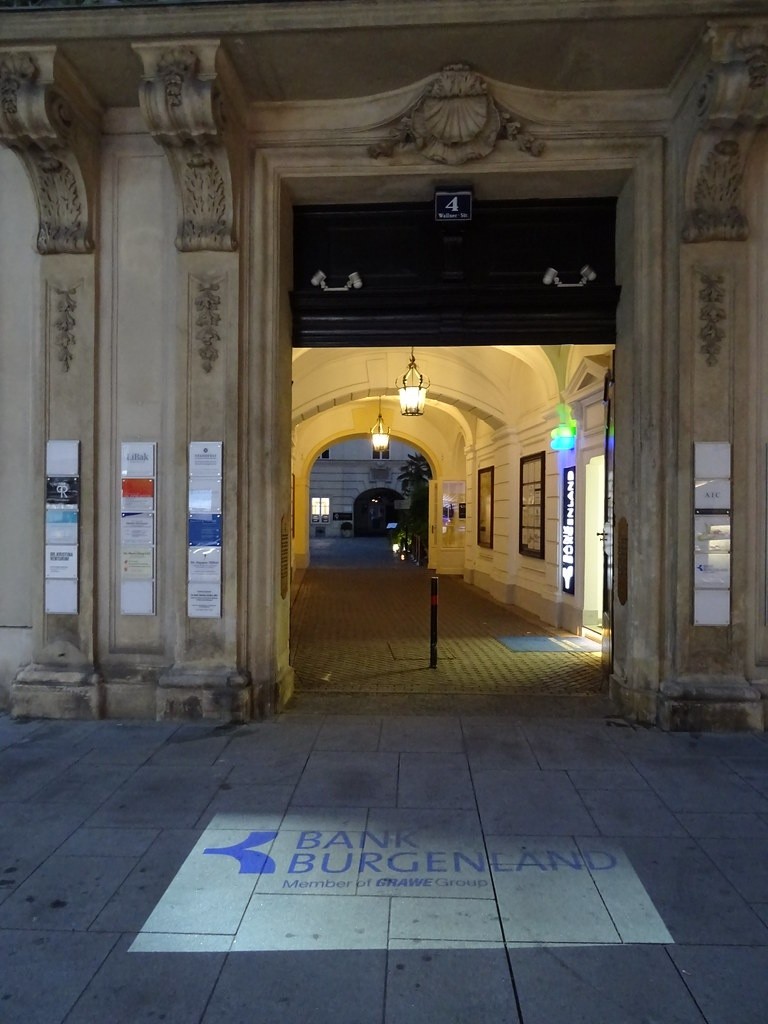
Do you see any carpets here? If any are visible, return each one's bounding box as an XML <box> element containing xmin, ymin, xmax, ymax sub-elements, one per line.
<box><xmin>496</xmin><ymin>634</ymin><xmax>601</xmax><ymax>653</ymax></box>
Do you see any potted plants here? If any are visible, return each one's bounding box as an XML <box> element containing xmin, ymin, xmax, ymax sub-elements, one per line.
<box><xmin>340</xmin><ymin>522</ymin><xmax>353</xmax><ymax>538</ymax></box>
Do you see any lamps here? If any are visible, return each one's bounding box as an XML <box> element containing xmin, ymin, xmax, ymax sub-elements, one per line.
<box><xmin>311</xmin><ymin>270</ymin><xmax>363</xmax><ymax>291</ymax></box>
<box><xmin>394</xmin><ymin>346</ymin><xmax>430</xmax><ymax>417</ymax></box>
<box><xmin>543</xmin><ymin>266</ymin><xmax>597</xmax><ymax>287</ymax></box>
<box><xmin>369</xmin><ymin>395</ymin><xmax>392</xmax><ymax>452</ymax></box>
